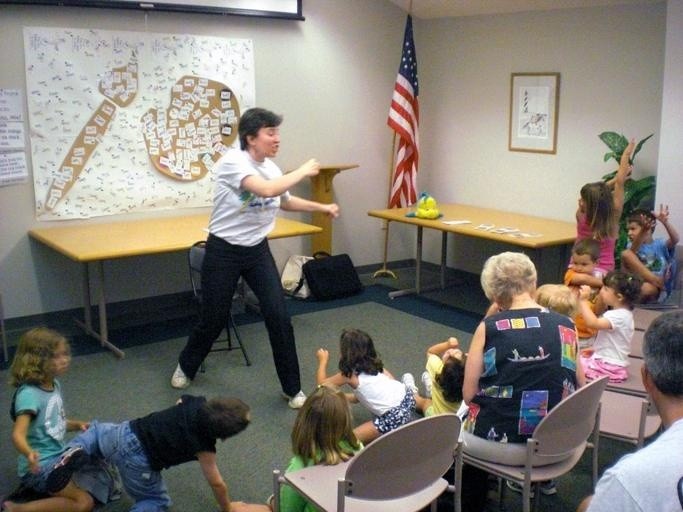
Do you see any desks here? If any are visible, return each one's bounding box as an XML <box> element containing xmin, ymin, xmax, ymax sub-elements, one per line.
<box><xmin>27</xmin><ymin>206</ymin><xmax>324</xmax><ymax>359</ymax></box>
<box><xmin>368</xmin><ymin>202</ymin><xmax>579</xmax><ymax>300</ymax></box>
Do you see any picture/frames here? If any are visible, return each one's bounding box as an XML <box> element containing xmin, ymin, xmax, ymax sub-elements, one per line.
<box><xmin>508</xmin><ymin>73</ymin><xmax>560</xmax><ymax>153</ymax></box>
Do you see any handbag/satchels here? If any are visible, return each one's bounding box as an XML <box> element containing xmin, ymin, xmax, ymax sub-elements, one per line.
<box><xmin>302</xmin><ymin>251</ymin><xmax>361</xmax><ymax>301</ymax></box>
<box><xmin>281</xmin><ymin>255</ymin><xmax>315</xmax><ymax>299</ymax></box>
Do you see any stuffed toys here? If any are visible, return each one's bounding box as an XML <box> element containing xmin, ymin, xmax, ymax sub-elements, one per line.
<box><xmin>404</xmin><ymin>191</ymin><xmax>443</xmax><ymax>220</ymax></box>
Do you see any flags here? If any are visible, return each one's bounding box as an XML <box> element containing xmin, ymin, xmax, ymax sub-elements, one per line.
<box><xmin>386</xmin><ymin>11</ymin><xmax>422</xmax><ymax>210</ymax></box>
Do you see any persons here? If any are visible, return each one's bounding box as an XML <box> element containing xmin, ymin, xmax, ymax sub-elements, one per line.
<box><xmin>44</xmin><ymin>388</ymin><xmax>269</xmax><ymax>512</ymax></box>
<box><xmin>2</xmin><ymin>325</ymin><xmax>97</xmax><ymax>512</ymax></box>
<box><xmin>227</xmin><ymin>381</ymin><xmax>368</xmax><ymax>512</ymax></box>
<box><xmin>170</xmin><ymin>101</ymin><xmax>345</xmax><ymax>412</ymax></box>
<box><xmin>566</xmin><ymin>137</ymin><xmax>637</xmax><ymax>278</ymax></box>
<box><xmin>312</xmin><ymin>328</ymin><xmax>418</xmax><ymax>441</ymax></box>
<box><xmin>444</xmin><ymin>202</ymin><xmax>683</xmax><ymax>512</ymax></box>
<box><xmin>398</xmin><ymin>333</ymin><xmax>467</xmax><ymax>422</ymax></box>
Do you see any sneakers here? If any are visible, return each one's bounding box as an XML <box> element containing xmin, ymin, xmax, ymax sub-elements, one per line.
<box><xmin>507</xmin><ymin>478</ymin><xmax>557</xmax><ymax>500</ymax></box>
<box><xmin>46</xmin><ymin>447</ymin><xmax>88</xmax><ymax>493</ymax></box>
<box><xmin>171</xmin><ymin>362</ymin><xmax>191</xmax><ymax>388</ymax></box>
<box><xmin>281</xmin><ymin>389</ymin><xmax>307</xmax><ymax>409</ymax></box>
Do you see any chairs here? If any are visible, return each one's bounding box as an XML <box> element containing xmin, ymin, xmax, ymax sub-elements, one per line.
<box><xmin>188</xmin><ymin>241</ymin><xmax>251</xmax><ymax>373</ymax></box>
